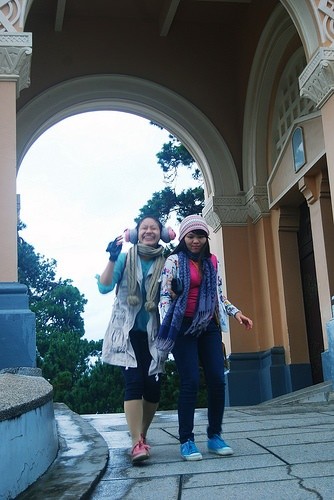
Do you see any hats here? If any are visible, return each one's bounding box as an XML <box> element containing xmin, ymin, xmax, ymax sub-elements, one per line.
<box><xmin>178</xmin><ymin>214</ymin><xmax>210</xmax><ymax>242</ymax></box>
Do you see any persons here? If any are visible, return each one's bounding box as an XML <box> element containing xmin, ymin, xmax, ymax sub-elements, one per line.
<box><xmin>97</xmin><ymin>216</ymin><xmax>176</xmax><ymax>462</ymax></box>
<box><xmin>148</xmin><ymin>215</ymin><xmax>253</xmax><ymax>460</ymax></box>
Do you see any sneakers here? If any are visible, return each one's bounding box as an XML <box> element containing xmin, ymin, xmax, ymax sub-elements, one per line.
<box><xmin>131</xmin><ymin>436</ymin><xmax>152</xmax><ymax>462</ymax></box>
<box><xmin>207</xmin><ymin>434</ymin><xmax>234</xmax><ymax>455</ymax></box>
<box><xmin>179</xmin><ymin>439</ymin><xmax>203</xmax><ymax>461</ymax></box>
